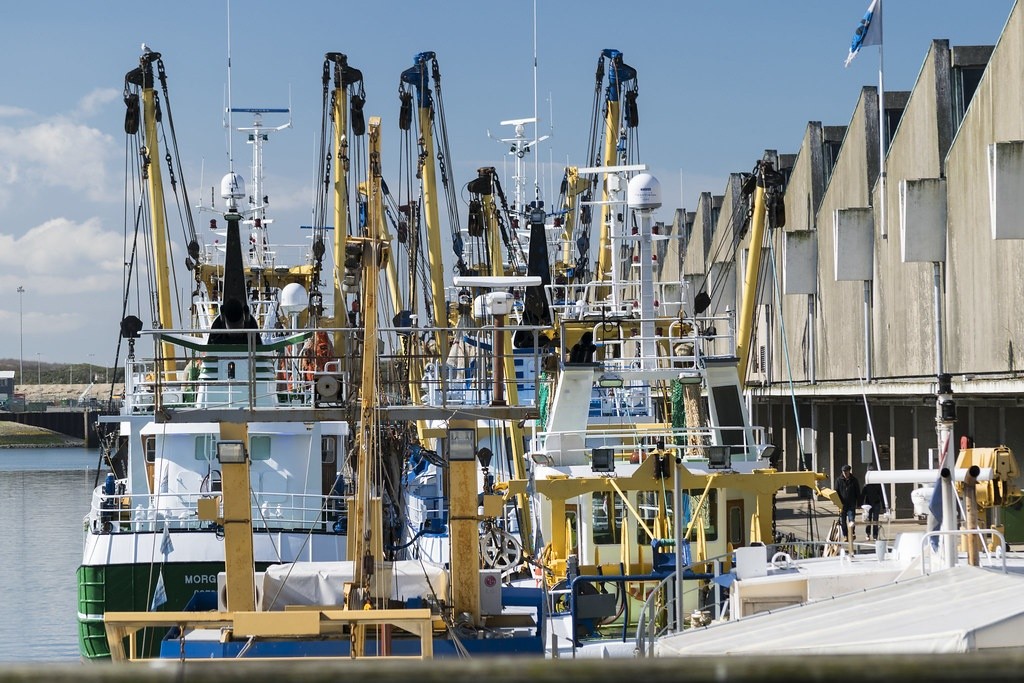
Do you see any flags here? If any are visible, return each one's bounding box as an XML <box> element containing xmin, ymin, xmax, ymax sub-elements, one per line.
<box><xmin>926</xmin><ymin>431</ymin><xmax>954</xmax><ymax>553</ymax></box>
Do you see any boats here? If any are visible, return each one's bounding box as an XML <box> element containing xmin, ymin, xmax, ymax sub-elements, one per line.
<box><xmin>75</xmin><ymin>3</ymin><xmax>762</xmax><ymax>658</ymax></box>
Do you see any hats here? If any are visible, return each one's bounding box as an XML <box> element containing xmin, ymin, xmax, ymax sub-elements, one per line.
<box><xmin>842</xmin><ymin>465</ymin><xmax>851</xmax><ymax>470</ymax></box>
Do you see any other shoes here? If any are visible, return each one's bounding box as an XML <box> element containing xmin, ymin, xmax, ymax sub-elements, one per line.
<box><xmin>853</xmin><ymin>535</ymin><xmax>856</xmax><ymax>541</ymax></box>
<box><xmin>865</xmin><ymin>536</ymin><xmax>870</xmax><ymax>540</ymax></box>
<box><xmin>843</xmin><ymin>537</ymin><xmax>848</xmax><ymax>542</ymax></box>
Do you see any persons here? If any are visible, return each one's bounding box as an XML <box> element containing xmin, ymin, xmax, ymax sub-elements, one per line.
<box><xmin>835</xmin><ymin>465</ymin><xmax>861</xmax><ymax>543</ymax></box>
<box><xmin>860</xmin><ymin>463</ymin><xmax>889</xmax><ymax>541</ymax></box>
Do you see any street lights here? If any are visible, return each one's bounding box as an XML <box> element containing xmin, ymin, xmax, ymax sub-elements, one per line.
<box><xmin>88</xmin><ymin>354</ymin><xmax>96</xmax><ymax>384</ymax></box>
<box><xmin>16</xmin><ymin>285</ymin><xmax>26</xmax><ymax>387</ymax></box>
<box><xmin>36</xmin><ymin>352</ymin><xmax>42</xmax><ymax>386</ymax></box>
